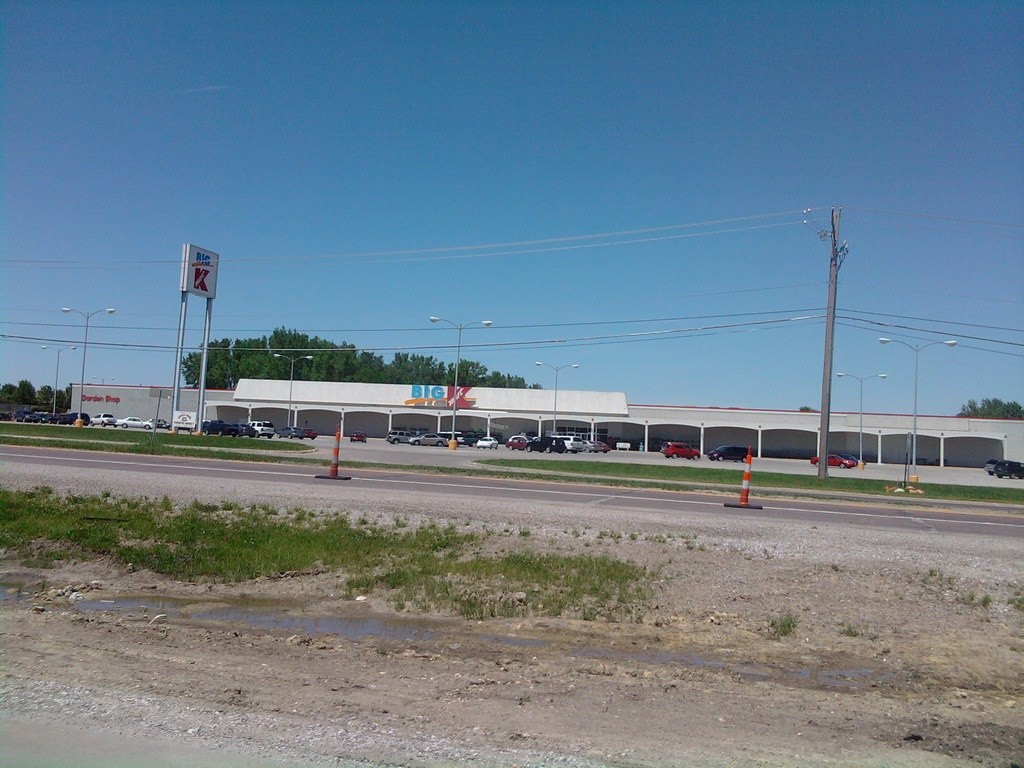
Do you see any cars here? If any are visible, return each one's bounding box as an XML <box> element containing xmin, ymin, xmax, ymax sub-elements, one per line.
<box><xmin>810</xmin><ymin>454</ymin><xmax>856</xmax><ymax>469</ymax></box>
<box><xmin>351</xmin><ymin>431</ymin><xmax>367</xmax><ymax>443</ymax></box>
<box><xmin>114</xmin><ymin>416</ymin><xmax>153</xmax><ymax>430</ymax></box>
<box><xmin>837</xmin><ymin>455</ymin><xmax>866</xmax><ymax>465</ymax></box>
<box><xmin>385</xmin><ymin>429</ymin><xmax>532</xmax><ymax>450</ymax></box>
<box><xmin>582</xmin><ymin>440</ymin><xmax>608</xmax><ymax>453</ymax></box>
<box><xmin>146</xmin><ymin>418</ymin><xmax>318</xmax><ymax>441</ymax></box>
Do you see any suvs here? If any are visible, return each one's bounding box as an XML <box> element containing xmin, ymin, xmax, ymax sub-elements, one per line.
<box><xmin>550</xmin><ymin>435</ymin><xmax>583</xmax><ymax>454</ymax></box>
<box><xmin>985</xmin><ymin>458</ymin><xmax>1024</xmax><ymax>480</ymax></box>
<box><xmin>15</xmin><ymin>410</ymin><xmax>117</xmax><ymax>427</ymax></box>
<box><xmin>662</xmin><ymin>442</ymin><xmax>700</xmax><ymax>461</ymax></box>
<box><xmin>525</xmin><ymin>437</ymin><xmax>565</xmax><ymax>454</ymax></box>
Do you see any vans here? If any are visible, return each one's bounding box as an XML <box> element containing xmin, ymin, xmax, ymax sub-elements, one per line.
<box><xmin>707</xmin><ymin>446</ymin><xmax>748</xmax><ymax>463</ymax></box>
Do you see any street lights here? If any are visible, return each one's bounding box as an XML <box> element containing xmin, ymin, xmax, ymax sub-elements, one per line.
<box><xmin>879</xmin><ymin>336</ymin><xmax>958</xmax><ymax>482</ymax></box>
<box><xmin>273</xmin><ymin>354</ymin><xmax>313</xmax><ymax>427</ymax></box>
<box><xmin>430</xmin><ymin>316</ymin><xmax>493</xmax><ymax>450</ymax></box>
<box><xmin>835</xmin><ymin>371</ymin><xmax>887</xmax><ymax>469</ymax></box>
<box><xmin>60</xmin><ymin>307</ymin><xmax>116</xmax><ymax>427</ymax></box>
<box><xmin>536</xmin><ymin>362</ymin><xmax>579</xmax><ymax>436</ymax></box>
<box><xmin>41</xmin><ymin>345</ymin><xmax>76</xmax><ymax>416</ymax></box>
